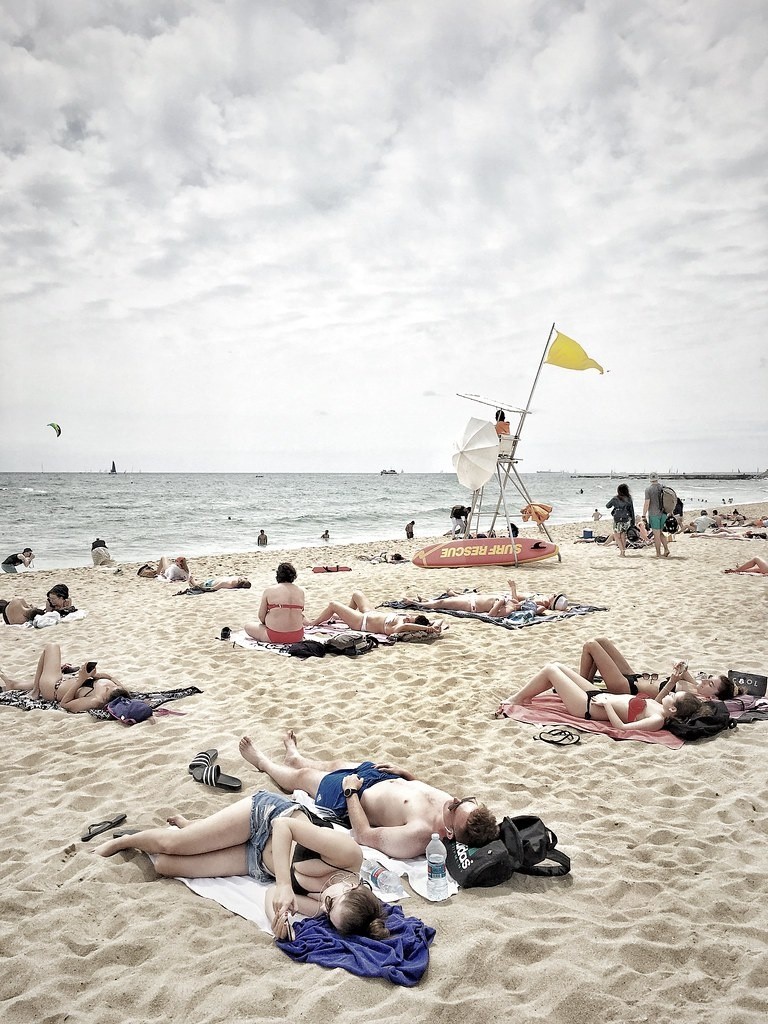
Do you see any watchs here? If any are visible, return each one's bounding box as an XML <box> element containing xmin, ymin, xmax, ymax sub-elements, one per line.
<box><xmin>343</xmin><ymin>787</ymin><xmax>358</xmax><ymax>798</ymax></box>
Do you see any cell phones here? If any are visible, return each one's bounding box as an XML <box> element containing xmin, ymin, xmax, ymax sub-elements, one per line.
<box><xmin>679</xmin><ymin>664</ymin><xmax>688</xmax><ymax>674</ymax></box>
<box><xmin>87</xmin><ymin>662</ymin><xmax>97</xmax><ymax>673</ymax></box>
<box><xmin>286</xmin><ymin>920</ymin><xmax>293</xmax><ymax>942</ymax></box>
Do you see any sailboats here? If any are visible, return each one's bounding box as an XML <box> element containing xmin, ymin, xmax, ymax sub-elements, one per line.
<box><xmin>109</xmin><ymin>461</ymin><xmax>118</xmax><ymax>474</ymax></box>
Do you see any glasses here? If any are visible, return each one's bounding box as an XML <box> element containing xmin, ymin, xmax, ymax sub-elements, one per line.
<box><xmin>554</xmin><ymin>594</ymin><xmax>557</xmax><ymax>598</ymax></box>
<box><xmin>448</xmin><ymin>796</ymin><xmax>478</xmax><ymax>840</ymax></box>
<box><xmin>325</xmin><ymin>890</ymin><xmax>352</xmax><ymax>920</ymax></box>
<box><xmin>636</xmin><ymin>673</ymin><xmax>658</xmax><ymax>684</ymax></box>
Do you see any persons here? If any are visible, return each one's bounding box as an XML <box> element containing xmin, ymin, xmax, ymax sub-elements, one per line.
<box><xmin>500</xmin><ymin>636</ymin><xmax>747</xmax><ymax>732</ymax></box>
<box><xmin>92</xmin><ymin>728</ymin><xmax>499</xmax><ymax>943</ymax></box>
<box><xmin>258</xmin><ymin>530</ymin><xmax>267</xmax><ymax>546</ymax></box>
<box><xmin>405</xmin><ymin>520</ymin><xmax>415</xmax><ymax>539</ymax></box>
<box><xmin>1</xmin><ymin>548</ymin><xmax>35</xmax><ymax>573</ymax></box>
<box><xmin>462</xmin><ymin>530</ymin><xmax>496</xmax><ymax>539</ymax></box>
<box><xmin>443</xmin><ymin>521</ymin><xmax>467</xmax><ymax>536</ymax></box>
<box><xmin>228</xmin><ymin>517</ymin><xmax>231</xmax><ymax>520</ymax></box>
<box><xmin>507</xmin><ymin>523</ymin><xmax>518</xmax><ymax>537</ymax></box>
<box><xmin>573</xmin><ymin>472</ymin><xmax>768</xmax><ymax>575</ymax></box>
<box><xmin>403</xmin><ymin>579</ymin><xmax>567</xmax><ymax>617</ymax></box>
<box><xmin>450</xmin><ymin>505</ymin><xmax>472</xmax><ymax>540</ymax></box>
<box><xmin>3</xmin><ymin>584</ymin><xmax>71</xmax><ymax>625</ymax></box>
<box><xmin>188</xmin><ymin>576</ymin><xmax>251</xmax><ymax>591</ymax></box>
<box><xmin>0</xmin><ymin>643</ymin><xmax>131</xmax><ymax>713</ymax></box>
<box><xmin>321</xmin><ymin>529</ymin><xmax>329</xmax><ymax>539</ymax></box>
<box><xmin>91</xmin><ymin>538</ymin><xmax>110</xmax><ymax>565</ymax></box>
<box><xmin>302</xmin><ymin>591</ymin><xmax>444</xmax><ymax>636</ymax></box>
<box><xmin>156</xmin><ymin>556</ymin><xmax>191</xmax><ymax>583</ymax></box>
<box><xmin>357</xmin><ymin>550</ymin><xmax>410</xmax><ymax>564</ymax></box>
<box><xmin>245</xmin><ymin>562</ymin><xmax>305</xmax><ymax>643</ymax></box>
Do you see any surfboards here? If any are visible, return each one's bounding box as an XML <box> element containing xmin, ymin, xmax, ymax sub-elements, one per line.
<box><xmin>412</xmin><ymin>538</ymin><xmax>559</xmax><ymax>569</ymax></box>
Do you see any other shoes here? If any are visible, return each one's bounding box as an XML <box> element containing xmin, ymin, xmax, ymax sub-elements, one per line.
<box><xmin>221</xmin><ymin>627</ymin><xmax>231</xmax><ymax>639</ymax></box>
<box><xmin>694</xmin><ymin>671</ymin><xmax>714</xmax><ymax>681</ymax></box>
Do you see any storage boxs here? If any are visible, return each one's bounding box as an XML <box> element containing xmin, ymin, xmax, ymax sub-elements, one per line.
<box><xmin>583</xmin><ymin>528</ymin><xmax>594</xmax><ymax>537</ymax></box>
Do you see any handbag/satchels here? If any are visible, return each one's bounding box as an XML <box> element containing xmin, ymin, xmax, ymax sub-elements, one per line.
<box><xmin>727</xmin><ymin>670</ymin><xmax>768</xmax><ymax>697</ymax></box>
<box><xmin>627</xmin><ymin>526</ymin><xmax>640</xmax><ymax>542</ymax></box>
<box><xmin>663</xmin><ymin>514</ymin><xmax>678</xmax><ymax>534</ymax></box>
<box><xmin>103</xmin><ymin>695</ymin><xmax>152</xmax><ymax>726</ymax></box>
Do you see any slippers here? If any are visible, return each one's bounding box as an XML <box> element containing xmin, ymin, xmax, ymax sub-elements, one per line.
<box><xmin>193</xmin><ymin>765</ymin><xmax>242</xmax><ymax>791</ymax></box>
<box><xmin>533</xmin><ymin>729</ymin><xmax>580</xmax><ymax>745</ymax></box>
<box><xmin>189</xmin><ymin>749</ymin><xmax>218</xmax><ymax>775</ymax></box>
<box><xmin>112</xmin><ymin>829</ymin><xmax>141</xmax><ymax>839</ymax></box>
<box><xmin>81</xmin><ymin>814</ymin><xmax>127</xmax><ymax>842</ymax></box>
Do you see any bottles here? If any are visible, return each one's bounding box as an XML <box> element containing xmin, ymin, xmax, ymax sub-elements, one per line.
<box><xmin>424</xmin><ymin>833</ymin><xmax>449</xmax><ymax>902</ymax></box>
<box><xmin>360</xmin><ymin>859</ymin><xmax>404</xmax><ymax>895</ymax></box>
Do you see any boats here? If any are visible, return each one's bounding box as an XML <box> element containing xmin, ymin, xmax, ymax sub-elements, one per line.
<box><xmin>384</xmin><ymin>470</ymin><xmax>398</xmax><ymax>474</ymax></box>
<box><xmin>411</xmin><ymin>535</ymin><xmax>560</xmax><ymax>569</ymax></box>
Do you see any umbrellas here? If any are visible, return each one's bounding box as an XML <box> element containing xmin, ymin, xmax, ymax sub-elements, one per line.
<box><xmin>452</xmin><ymin>417</ymin><xmax>499</xmax><ymax>490</ymax></box>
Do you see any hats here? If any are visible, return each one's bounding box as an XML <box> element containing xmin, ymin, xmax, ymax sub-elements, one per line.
<box><xmin>521</xmin><ymin>599</ymin><xmax>537</xmax><ymax>616</ymax></box>
<box><xmin>649</xmin><ymin>472</ymin><xmax>659</xmax><ymax>482</ymax></box>
<box><xmin>551</xmin><ymin>594</ymin><xmax>569</xmax><ymax>611</ymax></box>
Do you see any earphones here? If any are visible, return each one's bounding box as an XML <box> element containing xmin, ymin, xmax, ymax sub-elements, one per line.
<box><xmin>346</xmin><ymin>883</ymin><xmax>353</xmax><ymax>888</ymax></box>
<box><xmin>445</xmin><ymin>827</ymin><xmax>452</xmax><ymax>834</ymax></box>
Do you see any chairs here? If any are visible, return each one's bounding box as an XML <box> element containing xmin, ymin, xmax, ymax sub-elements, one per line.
<box><xmin>498</xmin><ymin>433</ymin><xmax>515</xmax><ymax>458</ymax></box>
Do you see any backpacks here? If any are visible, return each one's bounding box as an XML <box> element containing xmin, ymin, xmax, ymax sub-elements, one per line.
<box><xmin>662</xmin><ymin>700</ymin><xmax>737</xmax><ymax>741</ymax></box>
<box><xmin>613</xmin><ymin>497</ymin><xmax>629</xmax><ymax>522</ymax></box>
<box><xmin>658</xmin><ymin>483</ymin><xmax>677</xmax><ymax>514</ymax></box>
<box><xmin>327</xmin><ymin>632</ymin><xmax>379</xmax><ymax>654</ymax></box>
<box><xmin>442</xmin><ymin>815</ymin><xmax>571</xmax><ymax>889</ymax></box>
